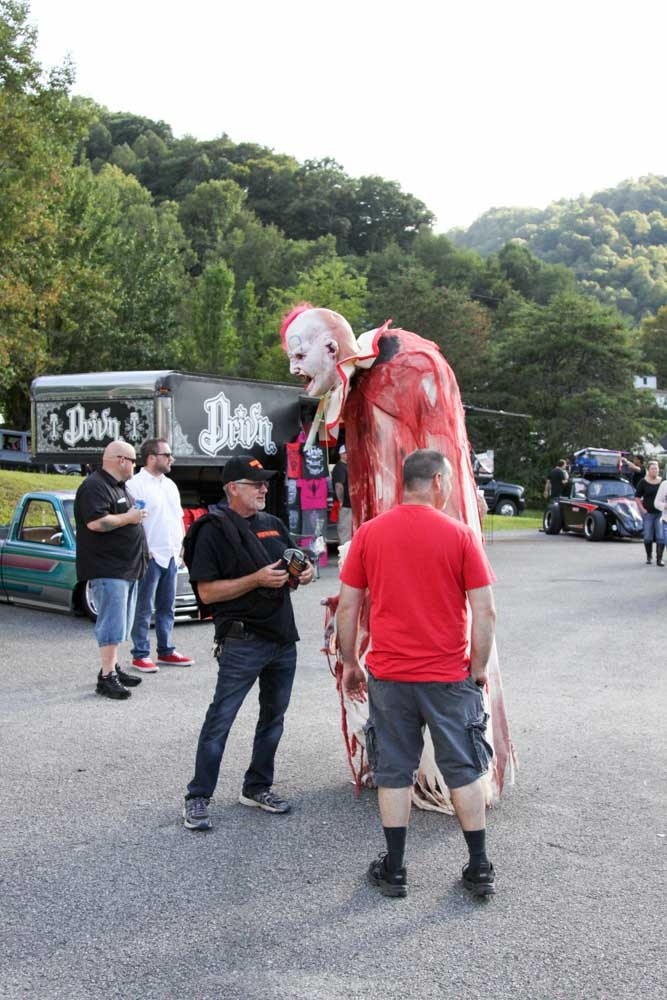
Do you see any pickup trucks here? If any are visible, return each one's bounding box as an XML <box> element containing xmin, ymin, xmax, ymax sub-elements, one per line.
<box><xmin>0</xmin><ymin>490</ymin><xmax>198</xmax><ymax>625</ymax></box>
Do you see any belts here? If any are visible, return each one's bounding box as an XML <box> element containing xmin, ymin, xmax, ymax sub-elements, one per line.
<box><xmin>224</xmin><ymin>632</ymin><xmax>260</xmax><ymax>642</ymax></box>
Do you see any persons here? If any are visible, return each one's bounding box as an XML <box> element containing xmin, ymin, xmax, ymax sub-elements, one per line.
<box><xmin>73</xmin><ymin>440</ymin><xmax>149</xmax><ymax>700</ymax></box>
<box><xmin>622</xmin><ymin>455</ymin><xmax>647</xmax><ymax>489</ymax></box>
<box><xmin>284</xmin><ymin>308</ymin><xmax>520</xmax><ymax>816</ymax></box>
<box><xmin>182</xmin><ymin>456</ymin><xmax>314</xmax><ymax>830</ymax></box>
<box><xmin>125</xmin><ymin>439</ymin><xmax>194</xmax><ymax>673</ymax></box>
<box><xmin>635</xmin><ymin>461</ymin><xmax>667</xmax><ymax>566</ymax></box>
<box><xmin>543</xmin><ymin>460</ymin><xmax>568</xmax><ymax>499</ymax></box>
<box><xmin>336</xmin><ymin>448</ymin><xmax>497</xmax><ymax>897</ymax></box>
<box><xmin>332</xmin><ymin>444</ymin><xmax>353</xmax><ymax>545</ymax></box>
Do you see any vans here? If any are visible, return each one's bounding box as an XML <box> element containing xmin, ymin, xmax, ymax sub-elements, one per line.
<box><xmin>0</xmin><ymin>430</ymin><xmax>89</xmax><ymax>476</ymax></box>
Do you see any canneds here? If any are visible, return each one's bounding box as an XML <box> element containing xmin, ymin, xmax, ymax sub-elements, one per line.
<box><xmin>134</xmin><ymin>500</ymin><xmax>145</xmax><ymax>509</ymax></box>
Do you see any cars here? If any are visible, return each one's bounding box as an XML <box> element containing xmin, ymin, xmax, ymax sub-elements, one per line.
<box><xmin>543</xmin><ymin>477</ymin><xmax>643</xmax><ymax>541</ymax></box>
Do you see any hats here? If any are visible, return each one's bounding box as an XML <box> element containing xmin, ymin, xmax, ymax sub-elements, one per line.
<box><xmin>222</xmin><ymin>455</ymin><xmax>280</xmax><ymax>483</ymax></box>
<box><xmin>339</xmin><ymin>444</ymin><xmax>347</xmax><ymax>454</ymax></box>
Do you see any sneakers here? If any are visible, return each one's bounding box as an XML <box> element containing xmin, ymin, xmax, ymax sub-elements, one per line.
<box><xmin>367</xmin><ymin>852</ymin><xmax>408</xmax><ymax>897</ymax></box>
<box><xmin>156</xmin><ymin>652</ymin><xmax>195</xmax><ymax>665</ymax></box>
<box><xmin>95</xmin><ymin>668</ymin><xmax>131</xmax><ymax>700</ymax></box>
<box><xmin>114</xmin><ymin>664</ymin><xmax>142</xmax><ymax>686</ymax></box>
<box><xmin>238</xmin><ymin>783</ymin><xmax>290</xmax><ymax>813</ymax></box>
<box><xmin>182</xmin><ymin>793</ymin><xmax>213</xmax><ymax>829</ymax></box>
<box><xmin>461</xmin><ymin>861</ymin><xmax>496</xmax><ymax>895</ymax></box>
<box><xmin>132</xmin><ymin>655</ymin><xmax>159</xmax><ymax>672</ymax></box>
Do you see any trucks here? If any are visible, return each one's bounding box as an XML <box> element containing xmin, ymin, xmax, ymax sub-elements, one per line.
<box><xmin>472</xmin><ymin>450</ymin><xmax>527</xmax><ymax>517</ymax></box>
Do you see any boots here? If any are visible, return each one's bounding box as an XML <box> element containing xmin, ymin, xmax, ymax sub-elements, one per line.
<box><xmin>657</xmin><ymin>544</ymin><xmax>665</xmax><ymax>565</ymax></box>
<box><xmin>644</xmin><ymin>543</ymin><xmax>652</xmax><ymax>563</ymax></box>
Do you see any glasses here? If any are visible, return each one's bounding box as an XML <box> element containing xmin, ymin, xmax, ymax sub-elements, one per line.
<box><xmin>117</xmin><ymin>455</ymin><xmax>136</xmax><ymax>463</ymax></box>
<box><xmin>154</xmin><ymin>453</ymin><xmax>173</xmax><ymax>458</ymax></box>
<box><xmin>234</xmin><ymin>481</ymin><xmax>269</xmax><ymax>489</ymax></box>
